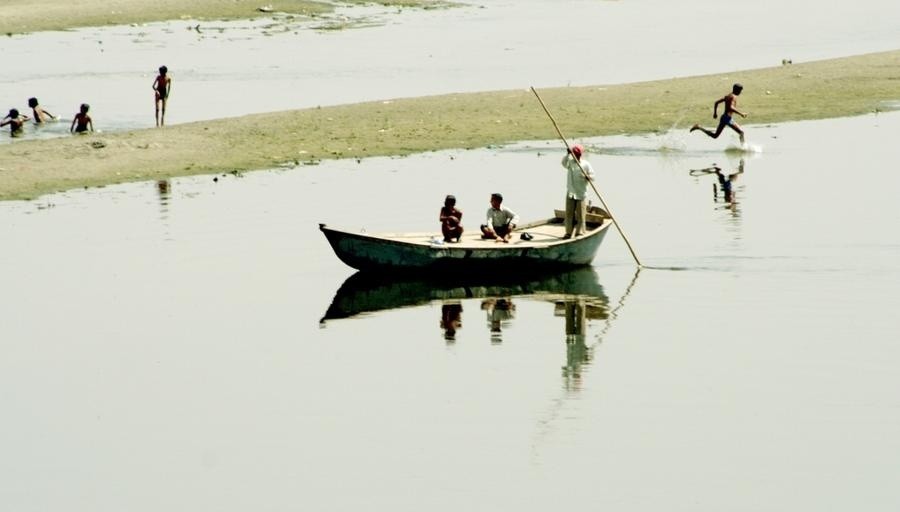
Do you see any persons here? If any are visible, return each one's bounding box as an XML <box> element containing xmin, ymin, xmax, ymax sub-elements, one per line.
<box><xmin>439</xmin><ymin>194</ymin><xmax>464</xmax><ymax>242</ymax></box>
<box><xmin>151</xmin><ymin>65</ymin><xmax>172</xmax><ymax>126</ymax></box>
<box><xmin>480</xmin><ymin>192</ymin><xmax>518</xmax><ymax>244</ymax></box>
<box><xmin>159</xmin><ymin>180</ymin><xmax>172</xmax><ymax>207</ymax></box>
<box><xmin>28</xmin><ymin>97</ymin><xmax>56</xmax><ymax>123</ymax></box>
<box><xmin>560</xmin><ymin>301</ymin><xmax>591</xmax><ymax>395</ymax></box>
<box><xmin>70</xmin><ymin>103</ymin><xmax>94</xmax><ymax>133</ymax></box>
<box><xmin>0</xmin><ymin>108</ymin><xmax>31</xmax><ymax>137</ymax></box>
<box><xmin>688</xmin><ymin>157</ymin><xmax>745</xmax><ymax>221</ymax></box>
<box><xmin>440</xmin><ymin>301</ymin><xmax>464</xmax><ymax>341</ymax></box>
<box><xmin>559</xmin><ymin>145</ymin><xmax>595</xmax><ymax>240</ymax></box>
<box><xmin>688</xmin><ymin>82</ymin><xmax>748</xmax><ymax>146</ymax></box>
<box><xmin>480</xmin><ymin>297</ymin><xmax>517</xmax><ymax>348</ymax></box>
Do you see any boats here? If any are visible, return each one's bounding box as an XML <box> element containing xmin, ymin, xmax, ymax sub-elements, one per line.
<box><xmin>319</xmin><ymin>200</ymin><xmax>613</xmax><ymax>270</ymax></box>
<box><xmin>319</xmin><ymin>271</ymin><xmax>610</xmax><ymax>329</ymax></box>
